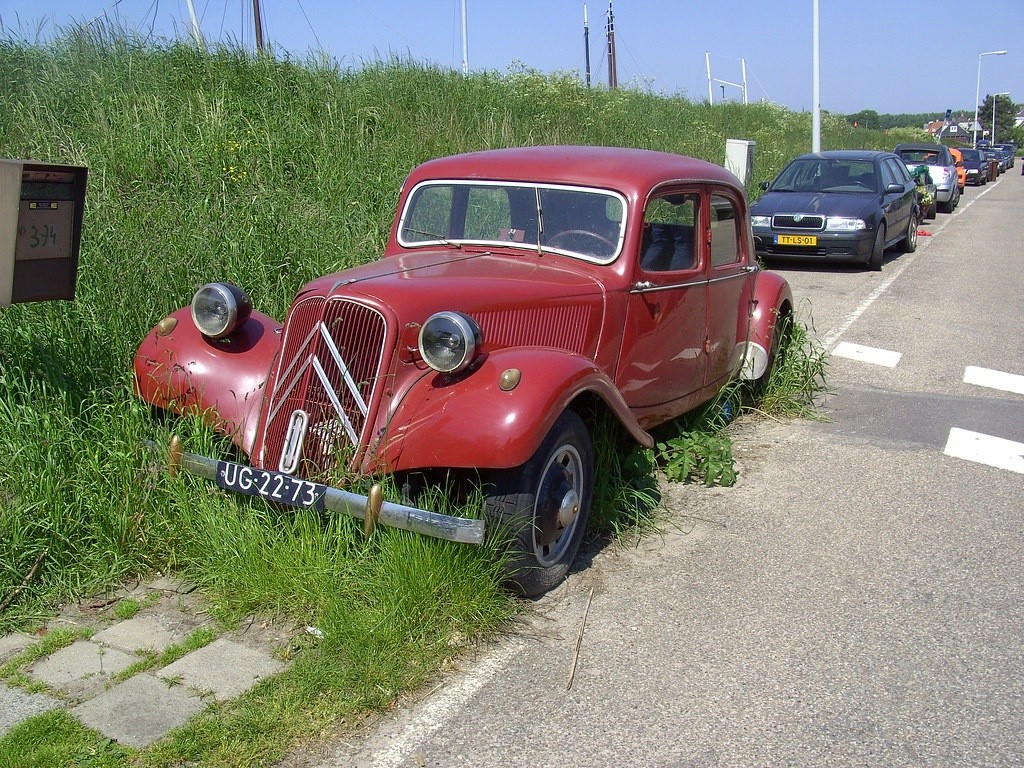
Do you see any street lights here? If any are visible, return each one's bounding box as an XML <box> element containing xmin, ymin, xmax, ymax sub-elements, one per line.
<box><xmin>973</xmin><ymin>50</ymin><xmax>1009</xmax><ymax>149</ymax></box>
<box><xmin>991</xmin><ymin>91</ymin><xmax>1012</xmax><ymax>147</ymax></box>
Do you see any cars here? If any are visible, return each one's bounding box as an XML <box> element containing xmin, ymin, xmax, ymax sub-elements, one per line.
<box><xmin>922</xmin><ymin>146</ymin><xmax>970</xmax><ymax>194</ymax></box>
<box><xmin>132</xmin><ymin>144</ymin><xmax>795</xmax><ymax>605</ymax></box>
<box><xmin>974</xmin><ymin>138</ymin><xmax>1017</xmax><ymax>182</ymax></box>
<box><xmin>748</xmin><ymin>149</ymin><xmax>925</xmax><ymax>271</ymax></box>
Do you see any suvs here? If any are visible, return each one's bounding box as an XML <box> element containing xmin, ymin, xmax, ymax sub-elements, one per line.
<box><xmin>888</xmin><ymin>142</ymin><xmax>964</xmax><ymax>214</ymax></box>
<box><xmin>951</xmin><ymin>147</ymin><xmax>990</xmax><ymax>187</ymax></box>
